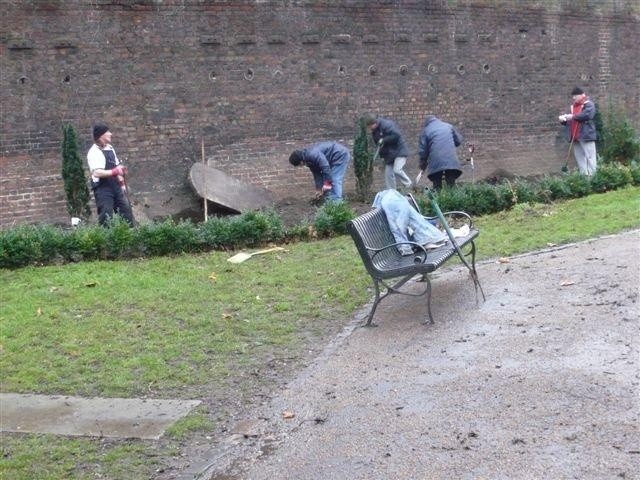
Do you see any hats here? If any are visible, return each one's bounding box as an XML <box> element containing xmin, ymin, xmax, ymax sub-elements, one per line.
<box><xmin>93</xmin><ymin>124</ymin><xmax>109</xmax><ymax>141</ymax></box>
<box><xmin>570</xmin><ymin>86</ymin><xmax>585</xmax><ymax>95</ymax></box>
<box><xmin>365</xmin><ymin>113</ymin><xmax>376</xmax><ymax>126</ymax></box>
<box><xmin>424</xmin><ymin>114</ymin><xmax>436</xmax><ymax>127</ymax></box>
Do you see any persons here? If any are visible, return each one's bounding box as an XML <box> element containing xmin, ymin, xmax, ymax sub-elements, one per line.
<box><xmin>363</xmin><ymin>113</ymin><xmax>412</xmax><ymax>190</ymax></box>
<box><xmin>88</xmin><ymin>125</ymin><xmax>136</xmax><ymax>226</ymax></box>
<box><xmin>418</xmin><ymin>116</ymin><xmax>464</xmax><ymax>192</ymax></box>
<box><xmin>289</xmin><ymin>141</ymin><xmax>351</xmax><ymax>203</ymax></box>
<box><xmin>558</xmin><ymin>89</ymin><xmax>597</xmax><ymax>176</ymax></box>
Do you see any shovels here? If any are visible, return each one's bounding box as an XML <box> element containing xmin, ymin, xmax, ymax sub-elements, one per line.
<box><xmin>561</xmin><ymin>105</ymin><xmax>585</xmax><ymax>173</ymax></box>
<box><xmin>226</xmin><ymin>246</ymin><xmax>284</xmax><ymax>263</ymax></box>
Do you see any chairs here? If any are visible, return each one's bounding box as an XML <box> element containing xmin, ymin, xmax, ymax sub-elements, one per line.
<box><xmin>349</xmin><ymin>192</ymin><xmax>480</xmax><ymax>325</ymax></box>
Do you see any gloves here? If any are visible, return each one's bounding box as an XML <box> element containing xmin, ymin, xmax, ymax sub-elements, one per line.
<box><xmin>558</xmin><ymin>114</ymin><xmax>565</xmax><ymax>123</ymax></box>
<box><xmin>111</xmin><ymin>164</ymin><xmax>127</xmax><ymax>178</ymax></box>
<box><xmin>565</xmin><ymin>113</ymin><xmax>574</xmax><ymax>123</ymax></box>
<box><xmin>321</xmin><ymin>182</ymin><xmax>332</xmax><ymax>193</ymax></box>
<box><xmin>314</xmin><ymin>191</ymin><xmax>324</xmax><ymax>201</ymax></box>
<box><xmin>117</xmin><ymin>178</ymin><xmax>131</xmax><ymax>195</ymax></box>
